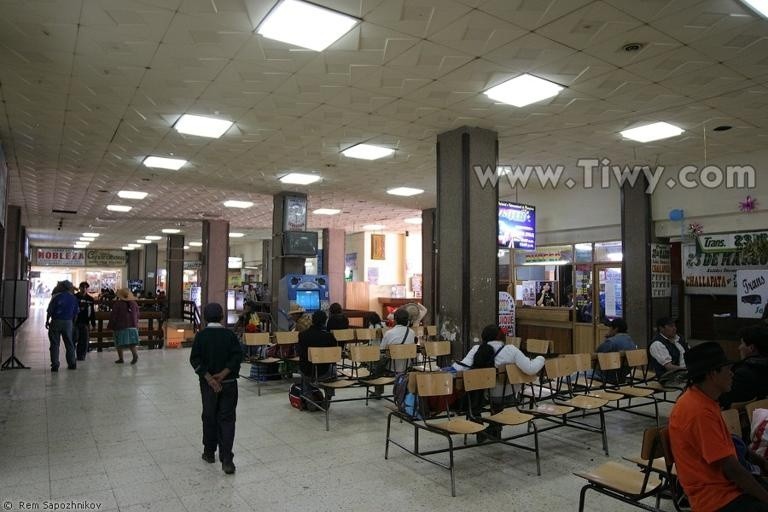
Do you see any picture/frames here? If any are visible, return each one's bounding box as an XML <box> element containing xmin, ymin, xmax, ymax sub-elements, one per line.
<box><xmin>371</xmin><ymin>234</ymin><xmax>385</xmax><ymax>260</ymax></box>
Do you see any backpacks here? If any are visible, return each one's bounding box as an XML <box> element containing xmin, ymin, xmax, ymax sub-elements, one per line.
<box><xmin>675</xmin><ymin>434</ymin><xmax>761</xmax><ymax>511</ymax></box>
<box><xmin>469</xmin><ymin>342</ymin><xmax>496</xmax><ymax>370</ymax></box>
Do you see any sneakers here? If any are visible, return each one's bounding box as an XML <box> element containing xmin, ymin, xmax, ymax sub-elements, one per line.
<box><xmin>115</xmin><ymin>358</ymin><xmax>124</xmax><ymax>363</ymax></box>
<box><xmin>323</xmin><ymin>399</ymin><xmax>330</xmax><ymax>410</ymax></box>
<box><xmin>131</xmin><ymin>354</ymin><xmax>138</xmax><ymax>363</ymax></box>
<box><xmin>222</xmin><ymin>455</ymin><xmax>236</xmax><ymax>473</ymax></box>
<box><xmin>202</xmin><ymin>449</ymin><xmax>215</xmax><ymax>463</ymax></box>
<box><xmin>51</xmin><ymin>367</ymin><xmax>58</xmax><ymax>371</ymax></box>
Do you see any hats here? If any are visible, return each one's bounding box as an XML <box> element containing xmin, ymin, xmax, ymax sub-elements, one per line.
<box><xmin>605</xmin><ymin>317</ymin><xmax>627</xmax><ymax>332</ymax></box>
<box><xmin>112</xmin><ymin>288</ymin><xmax>137</xmax><ymax>301</ymax></box>
<box><xmin>684</xmin><ymin>341</ymin><xmax>737</xmax><ymax>387</ymax></box>
<box><xmin>402</xmin><ymin>303</ymin><xmax>422</xmax><ymax>321</ymax></box>
<box><xmin>657</xmin><ymin>316</ymin><xmax>680</xmax><ymax>326</ymax></box>
<box><xmin>288</xmin><ymin>304</ymin><xmax>306</xmax><ymax>317</ymax></box>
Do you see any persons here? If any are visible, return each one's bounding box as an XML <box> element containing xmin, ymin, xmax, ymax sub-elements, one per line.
<box><xmin>536</xmin><ymin>281</ymin><xmax>557</xmax><ymax>307</ymax></box>
<box><xmin>188</xmin><ymin>302</ymin><xmax>243</xmax><ymax>475</ymax></box>
<box><xmin>668</xmin><ymin>340</ymin><xmax>768</xmax><ymax>512</ymax></box>
<box><xmin>647</xmin><ymin>319</ymin><xmax>692</xmax><ymax>384</ymax></box>
<box><xmin>716</xmin><ymin>325</ymin><xmax>768</xmax><ymax>411</ymax></box>
<box><xmin>565</xmin><ymin>284</ymin><xmax>578</xmax><ymax>307</ymax></box>
<box><xmin>559</xmin><ymin>317</ymin><xmax>637</xmax><ymax>385</ymax></box>
<box><xmin>451</xmin><ymin>324</ymin><xmax>546</xmax><ymax>443</ymax></box>
<box><xmin>579</xmin><ymin>293</ymin><xmax>606</xmax><ymax>322</ymax></box>
<box><xmin>230</xmin><ymin>276</ymin><xmax>238</xmax><ymax>285</ymax></box>
<box><xmin>45</xmin><ymin>280</ymin><xmax>428</xmax><ymax>400</ymax></box>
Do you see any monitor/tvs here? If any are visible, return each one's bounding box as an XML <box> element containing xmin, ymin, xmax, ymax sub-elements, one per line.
<box><xmin>292</xmin><ymin>288</ymin><xmax>322</xmax><ymax>313</ymax></box>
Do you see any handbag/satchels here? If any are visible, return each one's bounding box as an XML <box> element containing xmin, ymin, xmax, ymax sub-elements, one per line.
<box><xmin>267</xmin><ymin>343</ymin><xmax>297</xmax><ymax>357</ymax></box>
<box><xmin>364</xmin><ymin>357</ymin><xmax>390</xmax><ymax>375</ymax></box>
<box><xmin>393</xmin><ymin>372</ymin><xmax>485</xmax><ymax>419</ymax></box>
<box><xmin>660</xmin><ymin>367</ymin><xmax>687</xmax><ymax>390</ymax></box>
<box><xmin>289</xmin><ymin>383</ymin><xmax>323</xmax><ymax>411</ymax></box>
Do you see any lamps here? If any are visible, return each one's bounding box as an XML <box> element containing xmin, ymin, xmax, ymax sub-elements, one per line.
<box><xmin>0</xmin><ymin>280</ymin><xmax>31</xmax><ymax>370</ymax></box>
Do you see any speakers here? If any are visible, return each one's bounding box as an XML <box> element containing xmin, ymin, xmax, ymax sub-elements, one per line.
<box><xmin>282</xmin><ymin>230</ymin><xmax>319</xmax><ymax>257</ymax></box>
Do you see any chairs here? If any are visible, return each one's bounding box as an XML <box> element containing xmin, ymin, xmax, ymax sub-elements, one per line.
<box><xmin>244</xmin><ymin>317</ymin><xmax>767</xmax><ymax>512</ymax></box>
